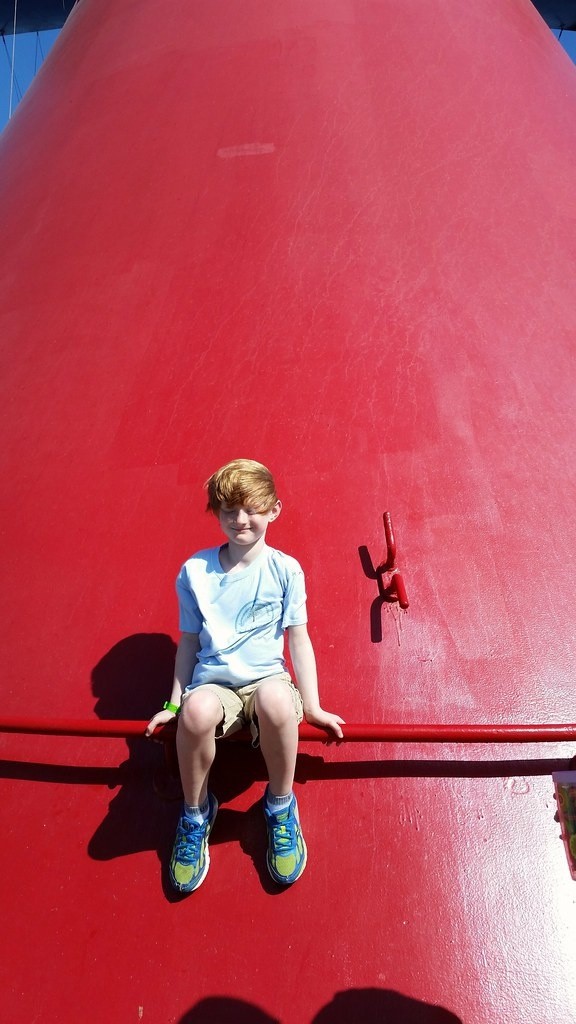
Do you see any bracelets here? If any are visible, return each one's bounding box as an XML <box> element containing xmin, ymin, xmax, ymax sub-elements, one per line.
<box><xmin>163</xmin><ymin>699</ymin><xmax>181</xmax><ymax>714</ymax></box>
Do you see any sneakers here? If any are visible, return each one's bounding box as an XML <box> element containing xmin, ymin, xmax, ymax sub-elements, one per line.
<box><xmin>262</xmin><ymin>783</ymin><xmax>307</xmax><ymax>884</ymax></box>
<box><xmin>169</xmin><ymin>790</ymin><xmax>218</xmax><ymax>893</ymax></box>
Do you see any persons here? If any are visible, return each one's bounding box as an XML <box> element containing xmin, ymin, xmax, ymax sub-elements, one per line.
<box><xmin>144</xmin><ymin>456</ymin><xmax>344</xmax><ymax>893</ymax></box>
